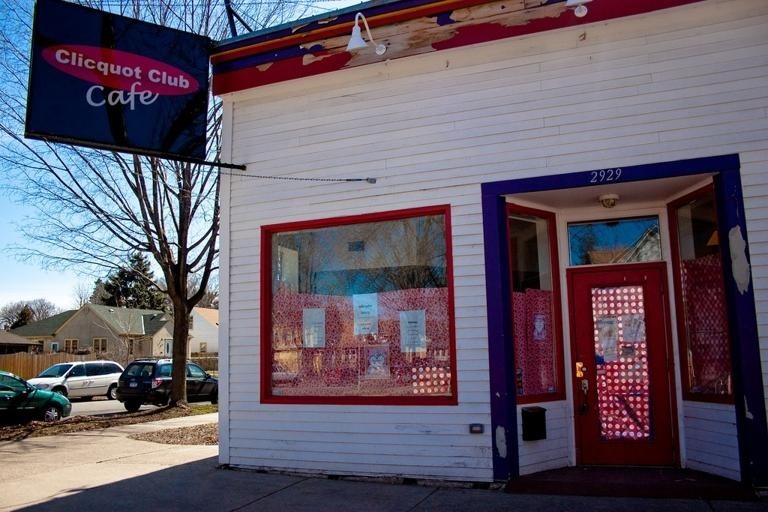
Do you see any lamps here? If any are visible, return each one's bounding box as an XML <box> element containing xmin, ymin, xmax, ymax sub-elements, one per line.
<box><xmin>599</xmin><ymin>194</ymin><xmax>619</xmax><ymax>208</ymax></box>
<box><xmin>565</xmin><ymin>0</ymin><xmax>592</xmax><ymax>17</ymax></box>
<box><xmin>346</xmin><ymin>12</ymin><xmax>386</xmax><ymax>55</ymax></box>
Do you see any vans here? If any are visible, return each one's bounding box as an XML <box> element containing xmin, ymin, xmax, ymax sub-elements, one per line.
<box><xmin>26</xmin><ymin>360</ymin><xmax>125</xmax><ymax>401</ymax></box>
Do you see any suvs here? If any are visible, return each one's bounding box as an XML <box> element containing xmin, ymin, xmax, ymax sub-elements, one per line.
<box><xmin>115</xmin><ymin>358</ymin><xmax>218</xmax><ymax>411</ymax></box>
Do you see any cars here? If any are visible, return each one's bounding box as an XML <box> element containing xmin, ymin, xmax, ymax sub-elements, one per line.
<box><xmin>0</xmin><ymin>370</ymin><xmax>72</xmax><ymax>421</ymax></box>
